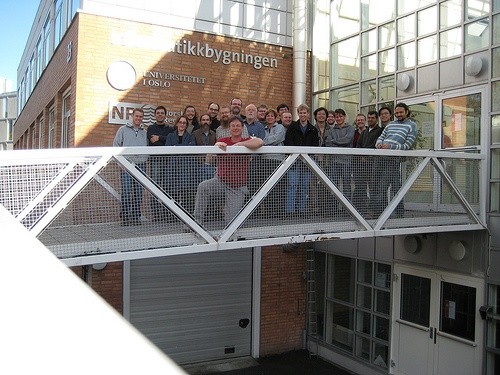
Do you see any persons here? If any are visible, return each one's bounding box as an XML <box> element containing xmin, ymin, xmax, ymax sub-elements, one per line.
<box><xmin>113</xmin><ymin>96</ymin><xmax>418</xmax><ymax>233</ymax></box>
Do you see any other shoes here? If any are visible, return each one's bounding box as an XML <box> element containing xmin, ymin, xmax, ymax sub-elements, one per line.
<box><xmin>121</xmin><ymin>219</ymin><xmax>142</xmax><ymax>226</ymax></box>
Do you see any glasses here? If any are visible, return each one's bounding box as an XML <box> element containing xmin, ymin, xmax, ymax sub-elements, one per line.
<box><xmin>210</xmin><ymin>108</ymin><xmax>219</xmax><ymax>111</ymax></box>
<box><xmin>202</xmin><ymin>119</ymin><xmax>211</xmax><ymax>121</ymax></box>
<box><xmin>179</xmin><ymin>121</ymin><xmax>187</xmax><ymax>124</ymax></box>
